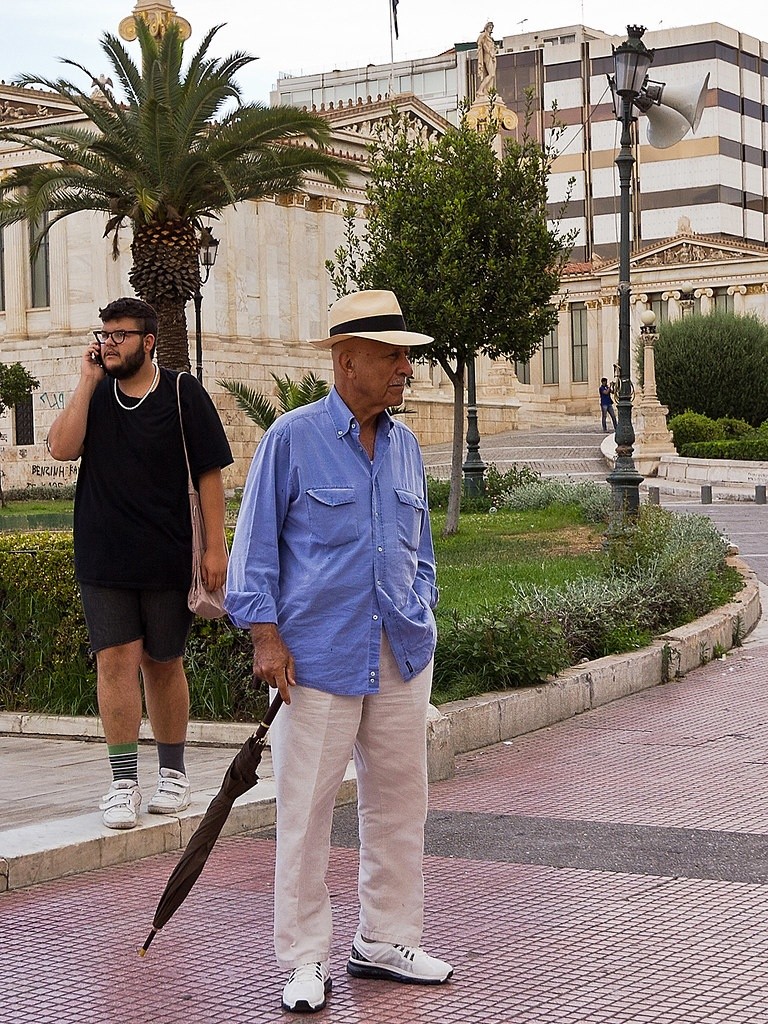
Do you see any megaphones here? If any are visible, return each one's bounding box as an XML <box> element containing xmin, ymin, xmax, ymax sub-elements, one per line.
<box><xmin>634</xmin><ymin>92</ymin><xmax>691</xmax><ymax>149</ymax></box>
<box><xmin>646</xmin><ymin>72</ymin><xmax>711</xmax><ymax>133</ymax></box>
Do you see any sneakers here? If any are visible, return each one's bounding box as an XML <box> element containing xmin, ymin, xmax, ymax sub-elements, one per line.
<box><xmin>99</xmin><ymin>779</ymin><xmax>142</xmax><ymax>828</ymax></box>
<box><xmin>148</xmin><ymin>767</ymin><xmax>191</xmax><ymax>813</ymax></box>
<box><xmin>281</xmin><ymin>958</ymin><xmax>332</xmax><ymax>1014</ymax></box>
<box><xmin>346</xmin><ymin>930</ymin><xmax>454</xmax><ymax>984</ymax></box>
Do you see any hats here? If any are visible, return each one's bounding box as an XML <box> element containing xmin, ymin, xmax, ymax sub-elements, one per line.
<box><xmin>307</xmin><ymin>289</ymin><xmax>434</xmax><ymax>350</ymax></box>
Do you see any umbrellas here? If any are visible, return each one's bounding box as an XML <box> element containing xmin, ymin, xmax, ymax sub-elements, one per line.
<box><xmin>138</xmin><ymin>679</ymin><xmax>292</xmax><ymax>954</ymax></box>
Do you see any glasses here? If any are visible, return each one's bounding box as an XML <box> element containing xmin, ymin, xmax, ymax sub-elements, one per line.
<box><xmin>93</xmin><ymin>329</ymin><xmax>145</xmax><ymax>344</ymax></box>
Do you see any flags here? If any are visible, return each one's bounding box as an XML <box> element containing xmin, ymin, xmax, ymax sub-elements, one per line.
<box><xmin>393</xmin><ymin>0</ymin><xmax>399</xmax><ymax>39</ymax></box>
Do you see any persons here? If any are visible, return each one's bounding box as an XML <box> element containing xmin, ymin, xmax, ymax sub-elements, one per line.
<box><xmin>345</xmin><ymin>113</ymin><xmax>445</xmax><ymax>144</ymax></box>
<box><xmin>590</xmin><ymin>250</ymin><xmax>604</xmax><ymax>262</ymax></box>
<box><xmin>46</xmin><ymin>297</ymin><xmax>236</xmax><ymax>830</ymax></box>
<box><xmin>224</xmin><ymin>288</ymin><xmax>451</xmax><ymax>1011</ymax></box>
<box><xmin>475</xmin><ymin>21</ymin><xmax>498</xmax><ymax>95</ymax></box>
<box><xmin>639</xmin><ymin>243</ymin><xmax>741</xmax><ymax>264</ymax></box>
<box><xmin>599</xmin><ymin>378</ymin><xmax>617</xmax><ymax>432</ymax></box>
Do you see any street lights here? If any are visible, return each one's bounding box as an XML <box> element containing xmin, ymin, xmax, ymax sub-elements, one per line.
<box><xmin>192</xmin><ymin>226</ymin><xmax>218</xmax><ymax>384</ymax></box>
<box><xmin>606</xmin><ymin>23</ymin><xmax>653</xmax><ymax>541</ymax></box>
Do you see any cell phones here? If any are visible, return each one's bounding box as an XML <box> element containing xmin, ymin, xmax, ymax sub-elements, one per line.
<box><xmin>96</xmin><ymin>349</ymin><xmax>106</xmax><ymax>374</ymax></box>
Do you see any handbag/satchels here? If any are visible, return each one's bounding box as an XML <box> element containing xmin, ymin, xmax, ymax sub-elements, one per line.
<box><xmin>190</xmin><ymin>490</ymin><xmax>230</xmax><ymax>619</ymax></box>
